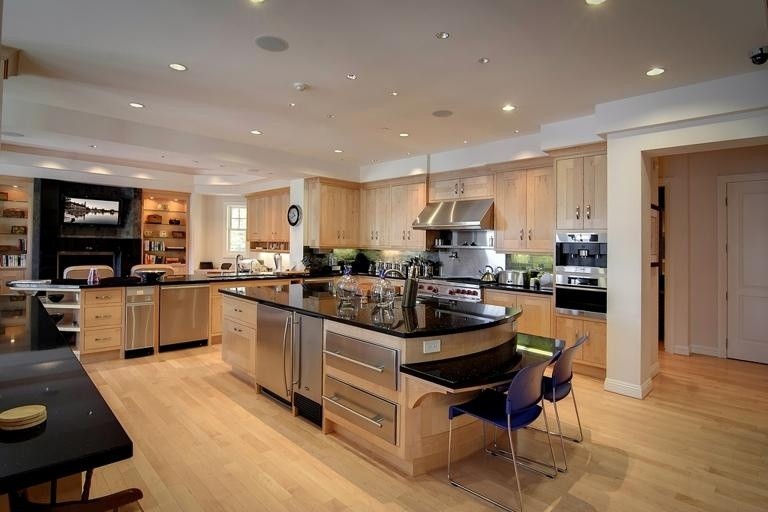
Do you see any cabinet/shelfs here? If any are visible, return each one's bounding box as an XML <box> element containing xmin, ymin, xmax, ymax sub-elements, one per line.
<box><xmin>427</xmin><ymin>174</ymin><xmax>497</xmax><ymax>201</ymax></box>
<box><xmin>360</xmin><ymin>179</ymin><xmax>393</xmax><ymax>253</ymax></box>
<box><xmin>554</xmin><ymin>313</ymin><xmax>606</xmax><ymax>382</ymax></box>
<box><xmin>553</xmin><ymin>153</ymin><xmax>606</xmax><ymax>235</ymax></box>
<box><xmin>479</xmin><ymin>288</ymin><xmax>555</xmax><ymax>367</ymax></box>
<box><xmin>266</xmin><ymin>191</ymin><xmax>294</xmax><ymax>253</ymax></box>
<box><xmin>79</xmin><ymin>288</ymin><xmax>122</xmax><ymax>363</ymax></box>
<box><xmin>391</xmin><ymin>178</ymin><xmax>429</xmax><ymax>251</ymax></box>
<box><xmin>245</xmin><ymin>192</ymin><xmax>269</xmax><ymax>253</ymax></box>
<box><xmin>493</xmin><ymin>162</ymin><xmax>556</xmax><ymax>256</ymax></box>
<box><xmin>220</xmin><ymin>292</ymin><xmax>259</xmax><ymax>389</ymax></box>
<box><xmin>321</xmin><ymin>176</ymin><xmax>363</xmax><ymax>250</ymax></box>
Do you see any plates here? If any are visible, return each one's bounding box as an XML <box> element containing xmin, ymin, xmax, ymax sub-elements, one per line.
<box><xmin>0</xmin><ymin>404</ymin><xmax>49</xmax><ymax>431</ymax></box>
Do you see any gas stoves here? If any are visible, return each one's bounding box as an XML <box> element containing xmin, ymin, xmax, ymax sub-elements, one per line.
<box><xmin>418</xmin><ymin>273</ymin><xmax>482</xmax><ymax>285</ymax></box>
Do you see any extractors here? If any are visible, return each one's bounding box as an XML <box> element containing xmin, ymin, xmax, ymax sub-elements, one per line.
<box><xmin>410</xmin><ymin>199</ymin><xmax>494</xmax><ymax>230</ymax></box>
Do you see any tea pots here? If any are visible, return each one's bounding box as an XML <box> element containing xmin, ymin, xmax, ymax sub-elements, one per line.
<box><xmin>478</xmin><ymin>265</ymin><xmax>496</xmax><ymax>281</ymax></box>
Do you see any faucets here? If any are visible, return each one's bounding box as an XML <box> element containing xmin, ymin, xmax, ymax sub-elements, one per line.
<box><xmin>235</xmin><ymin>253</ymin><xmax>244</xmax><ymax>276</ymax></box>
<box><xmin>381</xmin><ymin>269</ymin><xmax>406</xmax><ymax>279</ymax></box>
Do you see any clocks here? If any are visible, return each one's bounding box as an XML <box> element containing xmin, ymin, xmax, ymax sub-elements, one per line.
<box><xmin>288</xmin><ymin>205</ymin><xmax>303</xmax><ymax>228</ymax></box>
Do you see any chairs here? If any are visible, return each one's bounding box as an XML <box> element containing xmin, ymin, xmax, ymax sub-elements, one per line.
<box><xmin>492</xmin><ymin>330</ymin><xmax>593</xmax><ymax>473</ymax></box>
<box><xmin>446</xmin><ymin>349</ymin><xmax>563</xmax><ymax>511</ymax></box>
<box><xmin>10</xmin><ymin>486</ymin><xmax>152</xmax><ymax>512</ymax></box>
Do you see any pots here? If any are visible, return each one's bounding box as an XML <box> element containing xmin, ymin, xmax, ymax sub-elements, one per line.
<box><xmin>494</xmin><ymin>266</ymin><xmax>529</xmax><ymax>286</ymax></box>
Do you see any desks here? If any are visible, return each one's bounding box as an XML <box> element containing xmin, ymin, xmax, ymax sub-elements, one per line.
<box><xmin>396</xmin><ymin>331</ymin><xmax>569</xmax><ymax>408</ymax></box>
